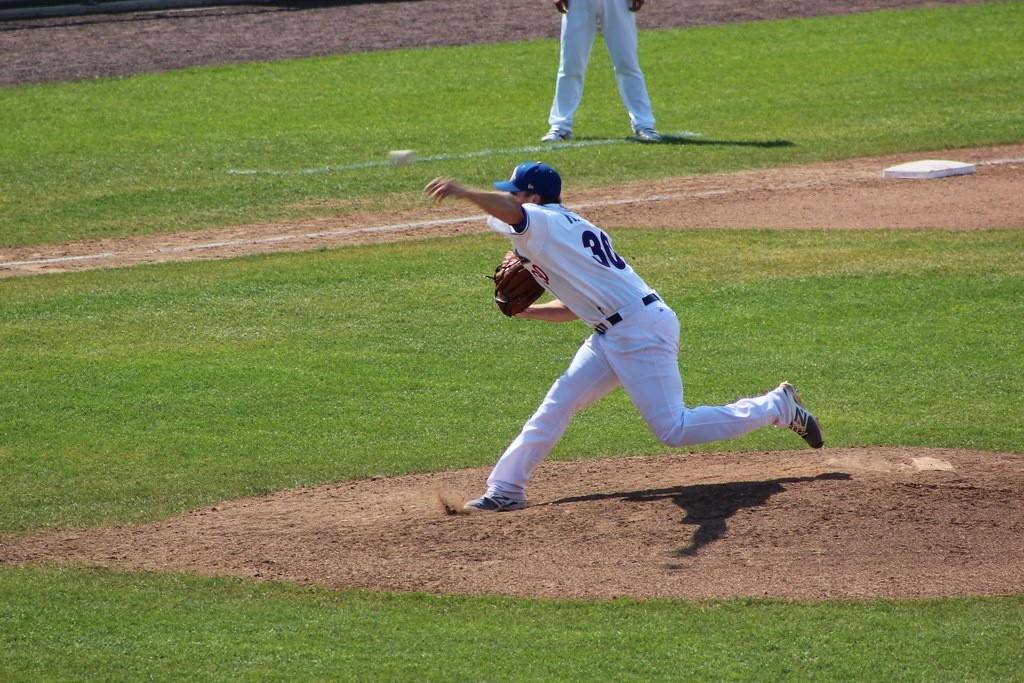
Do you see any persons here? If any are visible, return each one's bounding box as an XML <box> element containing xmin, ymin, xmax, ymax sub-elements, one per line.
<box><xmin>540</xmin><ymin>0</ymin><xmax>663</xmax><ymax>143</ymax></box>
<box><xmin>424</xmin><ymin>161</ymin><xmax>823</xmax><ymax>512</ymax></box>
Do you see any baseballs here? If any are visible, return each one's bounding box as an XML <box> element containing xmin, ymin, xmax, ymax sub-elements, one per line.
<box><xmin>388</xmin><ymin>149</ymin><xmax>414</xmax><ymax>165</ymax></box>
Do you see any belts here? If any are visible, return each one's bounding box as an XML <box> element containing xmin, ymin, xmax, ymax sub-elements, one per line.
<box><xmin>593</xmin><ymin>293</ymin><xmax>659</xmax><ymax>334</ymax></box>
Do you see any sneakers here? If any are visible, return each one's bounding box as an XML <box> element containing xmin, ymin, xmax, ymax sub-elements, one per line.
<box><xmin>636</xmin><ymin>127</ymin><xmax>661</xmax><ymax>143</ymax></box>
<box><xmin>462</xmin><ymin>488</ymin><xmax>526</xmax><ymax>514</ymax></box>
<box><xmin>778</xmin><ymin>380</ymin><xmax>824</xmax><ymax>448</ymax></box>
<box><xmin>541</xmin><ymin>127</ymin><xmax>572</xmax><ymax>142</ymax></box>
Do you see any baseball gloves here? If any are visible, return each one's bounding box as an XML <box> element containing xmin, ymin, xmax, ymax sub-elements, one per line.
<box><xmin>484</xmin><ymin>250</ymin><xmax>546</xmax><ymax>319</ymax></box>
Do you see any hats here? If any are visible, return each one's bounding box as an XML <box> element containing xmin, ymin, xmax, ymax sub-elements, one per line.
<box><xmin>494</xmin><ymin>161</ymin><xmax>561</xmax><ymax>197</ymax></box>
